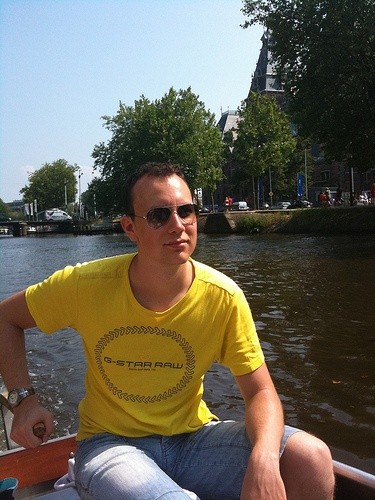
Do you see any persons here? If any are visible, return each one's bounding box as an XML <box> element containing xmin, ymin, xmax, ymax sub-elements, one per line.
<box><xmin>225</xmin><ymin>196</ymin><xmax>232</xmax><ymax>211</ymax></box>
<box><xmin>370</xmin><ymin>180</ymin><xmax>375</xmax><ymax>203</ymax></box>
<box><xmin>0</xmin><ymin>162</ymin><xmax>335</xmax><ymax>500</ymax></box>
<box><xmin>320</xmin><ymin>185</ymin><xmax>342</xmax><ymax>202</ymax></box>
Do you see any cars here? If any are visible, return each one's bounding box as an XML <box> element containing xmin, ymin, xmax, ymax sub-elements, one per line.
<box><xmin>286</xmin><ymin>199</ymin><xmax>310</xmax><ymax>209</ymax></box>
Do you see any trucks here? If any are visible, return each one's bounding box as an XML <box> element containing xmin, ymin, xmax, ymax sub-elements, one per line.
<box><xmin>231</xmin><ymin>201</ymin><xmax>247</xmax><ymax>211</ymax></box>
<box><xmin>45</xmin><ymin>210</ymin><xmax>72</xmax><ymax>221</ymax></box>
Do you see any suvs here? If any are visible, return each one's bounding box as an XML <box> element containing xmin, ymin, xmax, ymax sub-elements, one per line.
<box><xmin>271</xmin><ymin>202</ymin><xmax>291</xmax><ymax>209</ymax></box>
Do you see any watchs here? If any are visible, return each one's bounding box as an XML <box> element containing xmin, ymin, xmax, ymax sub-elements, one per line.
<box><xmin>8</xmin><ymin>387</ymin><xmax>35</xmax><ymax>407</ymax></box>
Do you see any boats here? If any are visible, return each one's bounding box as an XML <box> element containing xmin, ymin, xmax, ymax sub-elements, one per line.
<box><xmin>0</xmin><ymin>389</ymin><xmax>375</xmax><ymax>499</ymax></box>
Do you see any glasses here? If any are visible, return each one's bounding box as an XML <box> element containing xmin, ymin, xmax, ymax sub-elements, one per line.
<box><xmin>134</xmin><ymin>203</ymin><xmax>196</xmax><ymax>227</ymax></box>
